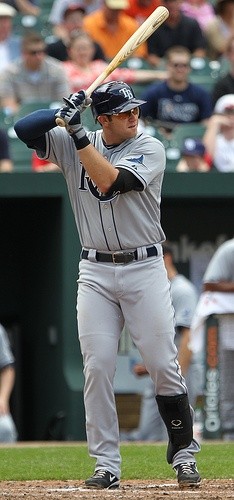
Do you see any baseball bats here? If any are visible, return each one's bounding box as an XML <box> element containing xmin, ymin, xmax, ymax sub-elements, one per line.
<box><xmin>56</xmin><ymin>5</ymin><xmax>169</xmax><ymax>130</ymax></box>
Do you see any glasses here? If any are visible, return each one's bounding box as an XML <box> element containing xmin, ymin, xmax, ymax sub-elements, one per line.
<box><xmin>172</xmin><ymin>63</ymin><xmax>188</xmax><ymax>69</ymax></box>
<box><xmin>26</xmin><ymin>49</ymin><xmax>46</xmax><ymax>56</ymax></box>
<box><xmin>101</xmin><ymin>107</ymin><xmax>139</xmax><ymax>120</ymax></box>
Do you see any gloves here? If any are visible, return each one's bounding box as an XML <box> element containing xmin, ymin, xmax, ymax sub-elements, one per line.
<box><xmin>67</xmin><ymin>89</ymin><xmax>92</xmax><ymax>113</ymax></box>
<box><xmin>54</xmin><ymin>97</ymin><xmax>87</xmax><ymax>142</ymax></box>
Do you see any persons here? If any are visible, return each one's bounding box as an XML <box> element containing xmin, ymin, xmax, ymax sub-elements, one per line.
<box><xmin>13</xmin><ymin>81</ymin><xmax>202</xmax><ymax>490</ymax></box>
<box><xmin>1</xmin><ymin>325</ymin><xmax>17</xmax><ymax>443</ymax></box>
<box><xmin>128</xmin><ymin>237</ymin><xmax>234</xmax><ymax>441</ymax></box>
<box><xmin>1</xmin><ymin>0</ymin><xmax>234</xmax><ymax>173</ymax></box>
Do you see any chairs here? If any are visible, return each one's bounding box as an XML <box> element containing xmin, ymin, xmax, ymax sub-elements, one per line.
<box><xmin>0</xmin><ymin>1</ymin><xmax>234</xmax><ymax>171</ymax></box>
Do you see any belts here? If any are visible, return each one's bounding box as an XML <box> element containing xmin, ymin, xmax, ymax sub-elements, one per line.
<box><xmin>81</xmin><ymin>246</ymin><xmax>157</xmax><ymax>263</ymax></box>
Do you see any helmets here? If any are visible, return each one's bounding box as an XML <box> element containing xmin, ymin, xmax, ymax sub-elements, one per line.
<box><xmin>90</xmin><ymin>80</ymin><xmax>147</xmax><ymax>124</ymax></box>
<box><xmin>214</xmin><ymin>94</ymin><xmax>234</xmax><ymax>115</ymax></box>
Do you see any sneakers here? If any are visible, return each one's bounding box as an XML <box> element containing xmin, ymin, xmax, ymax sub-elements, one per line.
<box><xmin>85</xmin><ymin>469</ymin><xmax>120</xmax><ymax>490</ymax></box>
<box><xmin>176</xmin><ymin>462</ymin><xmax>202</xmax><ymax>487</ymax></box>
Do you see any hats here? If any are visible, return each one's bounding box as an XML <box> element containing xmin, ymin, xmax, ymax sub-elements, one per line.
<box><xmin>61</xmin><ymin>1</ymin><xmax>85</xmax><ymax>18</ymax></box>
<box><xmin>0</xmin><ymin>3</ymin><xmax>16</xmax><ymax>18</ymax></box>
<box><xmin>180</xmin><ymin>138</ymin><xmax>205</xmax><ymax>157</ymax></box>
<box><xmin>103</xmin><ymin>0</ymin><xmax>130</xmax><ymax>10</ymax></box>
